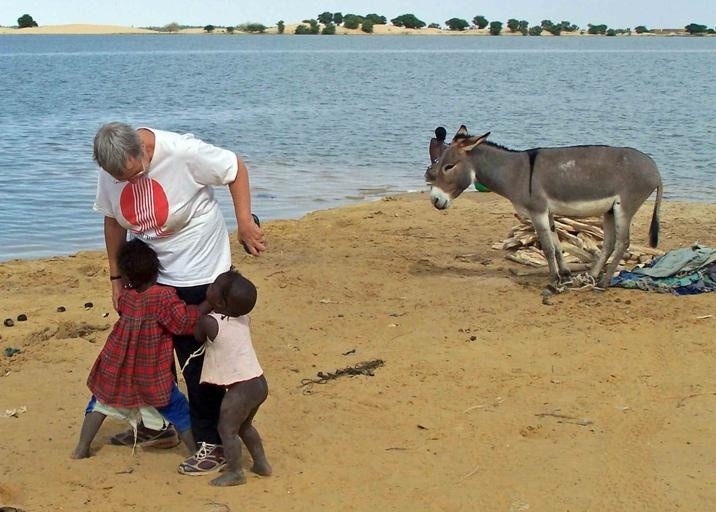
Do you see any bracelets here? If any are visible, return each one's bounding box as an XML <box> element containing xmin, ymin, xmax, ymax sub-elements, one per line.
<box><xmin>108</xmin><ymin>274</ymin><xmax>123</xmax><ymax>281</ymax></box>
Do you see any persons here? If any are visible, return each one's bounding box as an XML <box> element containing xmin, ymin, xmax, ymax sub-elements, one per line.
<box><xmin>192</xmin><ymin>267</ymin><xmax>274</xmax><ymax>486</ymax></box>
<box><xmin>429</xmin><ymin>126</ymin><xmax>451</xmax><ymax>162</ymax></box>
<box><xmin>92</xmin><ymin>120</ymin><xmax>267</xmax><ymax>478</ymax></box>
<box><xmin>68</xmin><ymin>238</ymin><xmax>197</xmax><ymax>458</ymax></box>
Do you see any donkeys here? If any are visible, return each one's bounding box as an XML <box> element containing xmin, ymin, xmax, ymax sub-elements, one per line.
<box><xmin>429</xmin><ymin>124</ymin><xmax>664</xmax><ymax>298</ymax></box>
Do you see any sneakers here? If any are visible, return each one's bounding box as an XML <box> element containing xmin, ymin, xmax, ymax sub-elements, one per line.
<box><xmin>110</xmin><ymin>422</ymin><xmax>179</xmax><ymax>449</ymax></box>
<box><xmin>176</xmin><ymin>442</ymin><xmax>229</xmax><ymax>477</ymax></box>
